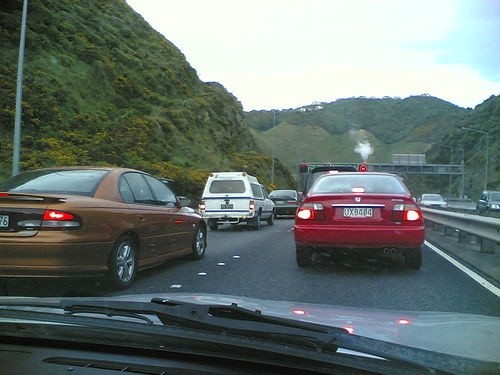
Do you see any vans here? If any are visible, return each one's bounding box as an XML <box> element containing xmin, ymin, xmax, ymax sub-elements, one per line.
<box><xmin>197</xmin><ymin>171</ymin><xmax>278</xmax><ymax>230</ymax></box>
<box><xmin>298</xmin><ymin>165</ymin><xmax>357</xmax><ymax>202</ymax></box>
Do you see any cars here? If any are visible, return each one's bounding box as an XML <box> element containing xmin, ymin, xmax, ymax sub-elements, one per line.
<box><xmin>267</xmin><ymin>189</ymin><xmax>302</xmax><ymax>218</ymax></box>
<box><xmin>0</xmin><ymin>164</ymin><xmax>208</xmax><ymax>291</ymax></box>
<box><xmin>420</xmin><ymin>194</ymin><xmax>449</xmax><ymax>209</ymax></box>
<box><xmin>293</xmin><ymin>169</ymin><xmax>426</xmax><ymax>266</ymax></box>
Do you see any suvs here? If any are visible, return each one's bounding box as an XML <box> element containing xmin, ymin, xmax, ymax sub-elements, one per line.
<box><xmin>475</xmin><ymin>190</ymin><xmax>500</xmax><ymax>218</ymax></box>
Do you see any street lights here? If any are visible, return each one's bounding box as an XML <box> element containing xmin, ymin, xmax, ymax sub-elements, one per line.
<box><xmin>271</xmin><ymin>109</ymin><xmax>293</xmax><ymax>188</ymax></box>
<box><xmin>459</xmin><ymin>126</ymin><xmax>490</xmax><ymax>190</ymax></box>
